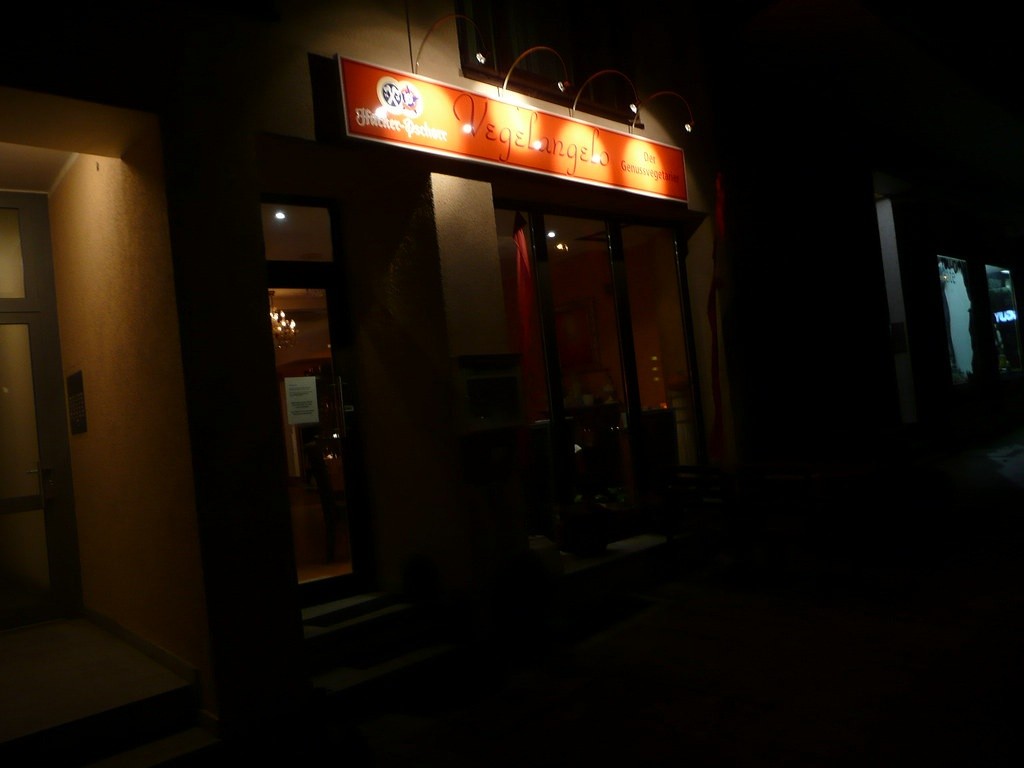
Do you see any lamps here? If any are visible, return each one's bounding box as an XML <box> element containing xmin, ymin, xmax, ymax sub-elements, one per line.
<box><xmin>571</xmin><ymin>68</ymin><xmax>641</xmax><ymax>118</ymax></box>
<box><xmin>631</xmin><ymin>90</ymin><xmax>694</xmax><ymax>137</ymax></box>
<box><xmin>267</xmin><ymin>288</ymin><xmax>297</xmax><ymax>349</ymax></box>
<box><xmin>501</xmin><ymin>44</ymin><xmax>569</xmax><ymax>100</ymax></box>
<box><xmin>415</xmin><ymin>12</ymin><xmax>488</xmax><ymax>74</ymax></box>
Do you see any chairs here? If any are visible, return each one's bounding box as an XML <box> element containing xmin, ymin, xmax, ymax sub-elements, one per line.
<box><xmin>305</xmin><ymin>440</ymin><xmax>346</xmax><ymax>565</ymax></box>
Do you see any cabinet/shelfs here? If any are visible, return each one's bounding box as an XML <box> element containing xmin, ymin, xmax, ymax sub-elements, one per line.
<box><xmin>278</xmin><ymin>356</ymin><xmax>342</xmax><ymax>493</ymax></box>
